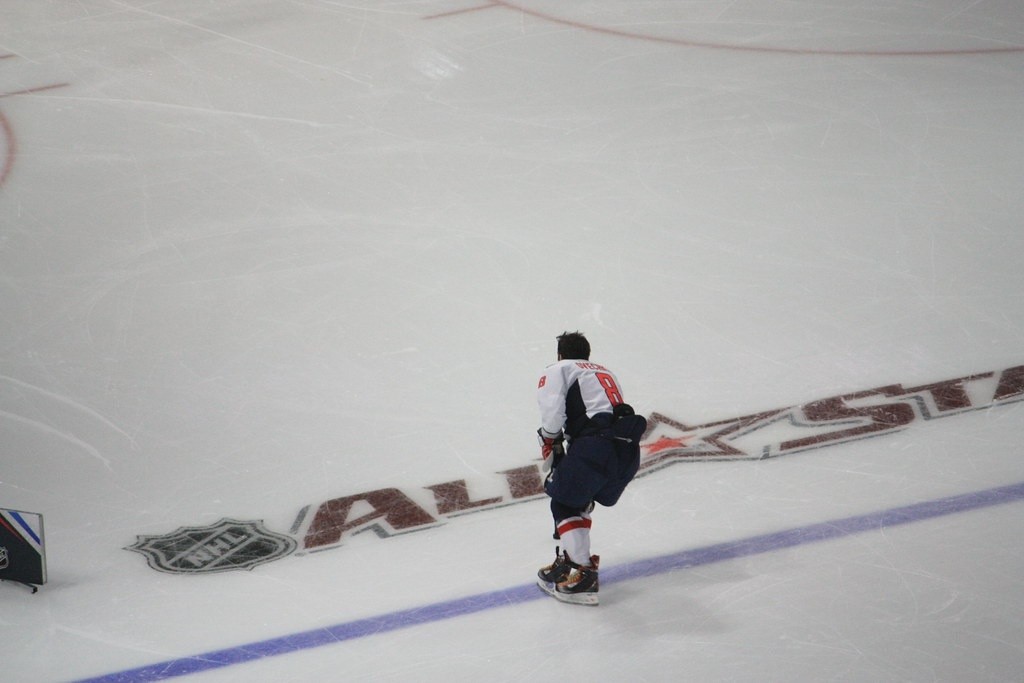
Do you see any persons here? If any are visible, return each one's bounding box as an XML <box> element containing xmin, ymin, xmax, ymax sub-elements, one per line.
<box><xmin>536</xmin><ymin>331</ymin><xmax>639</xmax><ymax>595</ymax></box>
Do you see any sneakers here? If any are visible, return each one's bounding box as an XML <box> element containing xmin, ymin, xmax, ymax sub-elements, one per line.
<box><xmin>538</xmin><ymin>546</ymin><xmax>582</xmax><ymax>596</ymax></box>
<box><xmin>553</xmin><ymin>554</ymin><xmax>599</xmax><ymax>606</ymax></box>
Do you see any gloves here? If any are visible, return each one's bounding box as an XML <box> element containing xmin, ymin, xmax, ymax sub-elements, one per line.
<box><xmin>537</xmin><ymin>428</ymin><xmax>565</xmax><ymax>467</ymax></box>
